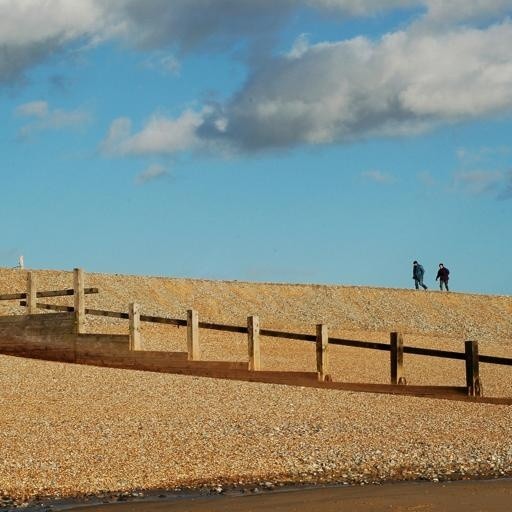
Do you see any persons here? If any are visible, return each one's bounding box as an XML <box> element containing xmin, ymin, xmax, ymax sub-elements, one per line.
<box><xmin>412</xmin><ymin>260</ymin><xmax>429</xmax><ymax>291</ymax></box>
<box><xmin>435</xmin><ymin>263</ymin><xmax>450</xmax><ymax>291</ymax></box>
